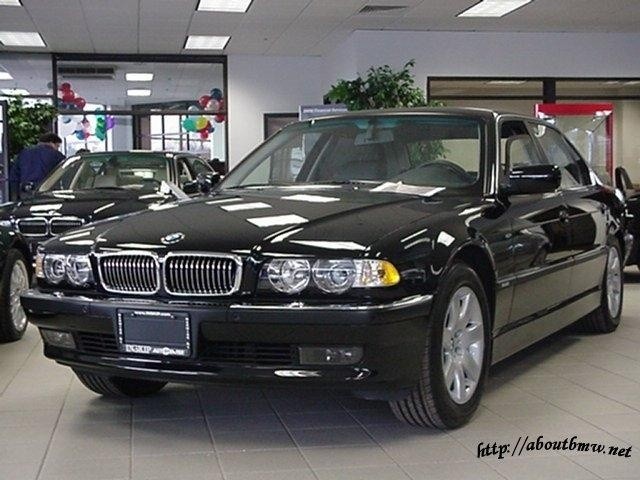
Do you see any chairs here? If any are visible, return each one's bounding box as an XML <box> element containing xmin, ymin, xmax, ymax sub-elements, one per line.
<box><xmin>84</xmin><ymin>166</ymin><xmax>166</xmax><ymax>185</ymax></box>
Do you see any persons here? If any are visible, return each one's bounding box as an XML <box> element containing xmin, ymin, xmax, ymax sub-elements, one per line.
<box><xmin>10</xmin><ymin>132</ymin><xmax>67</xmax><ymax>199</ymax></box>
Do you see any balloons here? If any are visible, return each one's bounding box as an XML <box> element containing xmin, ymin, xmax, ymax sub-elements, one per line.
<box><xmin>57</xmin><ymin>83</ymin><xmax>115</xmax><ymax>142</ymax></box>
<box><xmin>181</xmin><ymin>88</ymin><xmax>226</xmax><ymax>141</ymax></box>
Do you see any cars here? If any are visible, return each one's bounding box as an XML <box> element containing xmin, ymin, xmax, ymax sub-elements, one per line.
<box><xmin>0</xmin><ymin>225</ymin><xmax>33</xmax><ymax>343</ymax></box>
<box><xmin>19</xmin><ymin>106</ymin><xmax>634</xmax><ymax>429</ymax></box>
<box><xmin>9</xmin><ymin>150</ymin><xmax>218</xmax><ymax>274</ymax></box>
